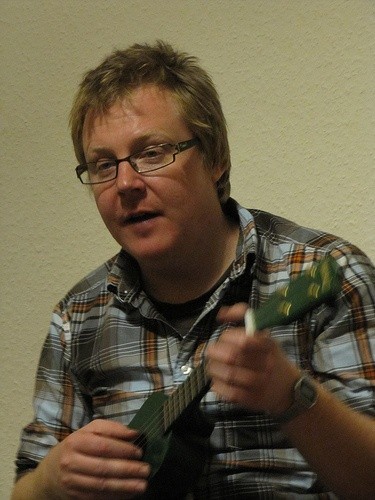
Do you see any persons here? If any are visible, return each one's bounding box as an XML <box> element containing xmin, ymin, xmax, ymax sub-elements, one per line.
<box><xmin>11</xmin><ymin>40</ymin><xmax>375</xmax><ymax>500</ymax></box>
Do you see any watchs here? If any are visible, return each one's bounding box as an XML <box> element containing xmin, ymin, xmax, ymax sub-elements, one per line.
<box><xmin>274</xmin><ymin>375</ymin><xmax>319</xmax><ymax>423</ymax></box>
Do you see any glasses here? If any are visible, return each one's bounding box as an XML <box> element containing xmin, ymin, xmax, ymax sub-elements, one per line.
<box><xmin>75</xmin><ymin>136</ymin><xmax>199</xmax><ymax>185</ymax></box>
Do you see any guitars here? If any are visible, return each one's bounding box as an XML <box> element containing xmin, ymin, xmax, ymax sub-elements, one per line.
<box><xmin>127</xmin><ymin>252</ymin><xmax>344</xmax><ymax>500</ymax></box>
